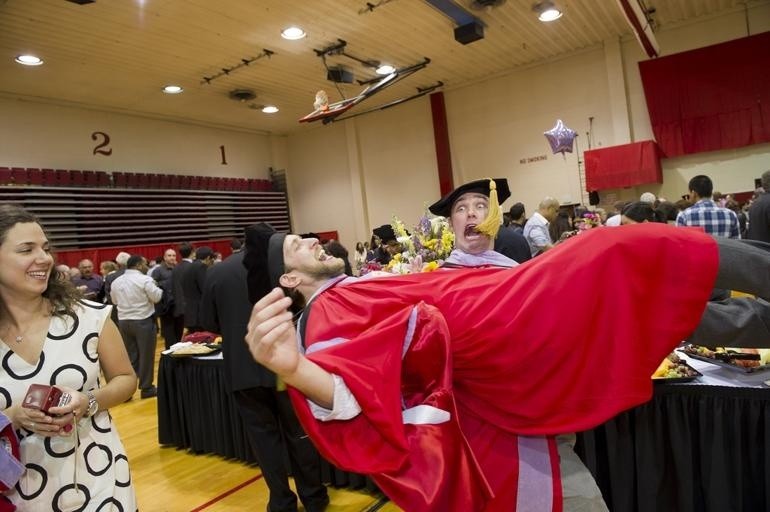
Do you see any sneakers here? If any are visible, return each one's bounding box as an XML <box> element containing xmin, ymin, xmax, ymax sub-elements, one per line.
<box><xmin>140</xmin><ymin>384</ymin><xmax>159</xmax><ymax>399</ymax></box>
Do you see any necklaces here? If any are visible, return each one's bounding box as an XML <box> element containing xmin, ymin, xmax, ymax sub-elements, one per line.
<box><xmin>6</xmin><ymin>322</ymin><xmax>35</xmax><ymax>343</ymax></box>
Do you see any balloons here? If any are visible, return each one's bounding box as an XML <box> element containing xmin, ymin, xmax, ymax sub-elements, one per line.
<box><xmin>543</xmin><ymin>118</ymin><xmax>576</xmax><ymax>157</ymax></box>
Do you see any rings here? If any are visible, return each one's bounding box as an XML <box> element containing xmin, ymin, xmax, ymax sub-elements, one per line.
<box><xmin>30</xmin><ymin>421</ymin><xmax>36</xmax><ymax>430</ymax></box>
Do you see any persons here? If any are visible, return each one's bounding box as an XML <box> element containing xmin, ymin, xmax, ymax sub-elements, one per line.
<box><xmin>1</xmin><ymin>202</ymin><xmax>138</xmax><ymax>512</ymax></box>
<box><xmin>240</xmin><ymin>224</ymin><xmax>769</xmax><ymax>510</ymax></box>
<box><xmin>304</xmin><ymin>225</ymin><xmax>404</xmax><ymax>277</ymax></box>
<box><xmin>494</xmin><ymin>171</ymin><xmax>770</xmax><ymax>303</ymax></box>
<box><xmin>56</xmin><ymin>238</ymin><xmax>245</xmax><ymax>402</ymax></box>
<box><xmin>198</xmin><ymin>245</ymin><xmax>330</xmax><ymax>512</ymax></box>
<box><xmin>423</xmin><ymin>176</ymin><xmax>608</xmax><ymax>510</ymax></box>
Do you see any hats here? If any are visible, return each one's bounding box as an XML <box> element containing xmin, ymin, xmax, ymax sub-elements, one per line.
<box><xmin>240</xmin><ymin>218</ymin><xmax>289</xmax><ymax>307</ymax></box>
<box><xmin>425</xmin><ymin>175</ymin><xmax>512</xmax><ymax>217</ymax></box>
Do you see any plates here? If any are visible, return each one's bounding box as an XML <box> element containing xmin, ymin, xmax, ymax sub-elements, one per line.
<box><xmin>648</xmin><ymin>347</ymin><xmax>769</xmax><ymax>385</ymax></box>
<box><xmin>169</xmin><ymin>344</ymin><xmax>220</xmax><ymax>358</ymax></box>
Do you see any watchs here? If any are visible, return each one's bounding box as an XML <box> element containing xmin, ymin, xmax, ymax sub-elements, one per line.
<box><xmin>87</xmin><ymin>390</ymin><xmax>98</xmax><ymax>417</ymax></box>
